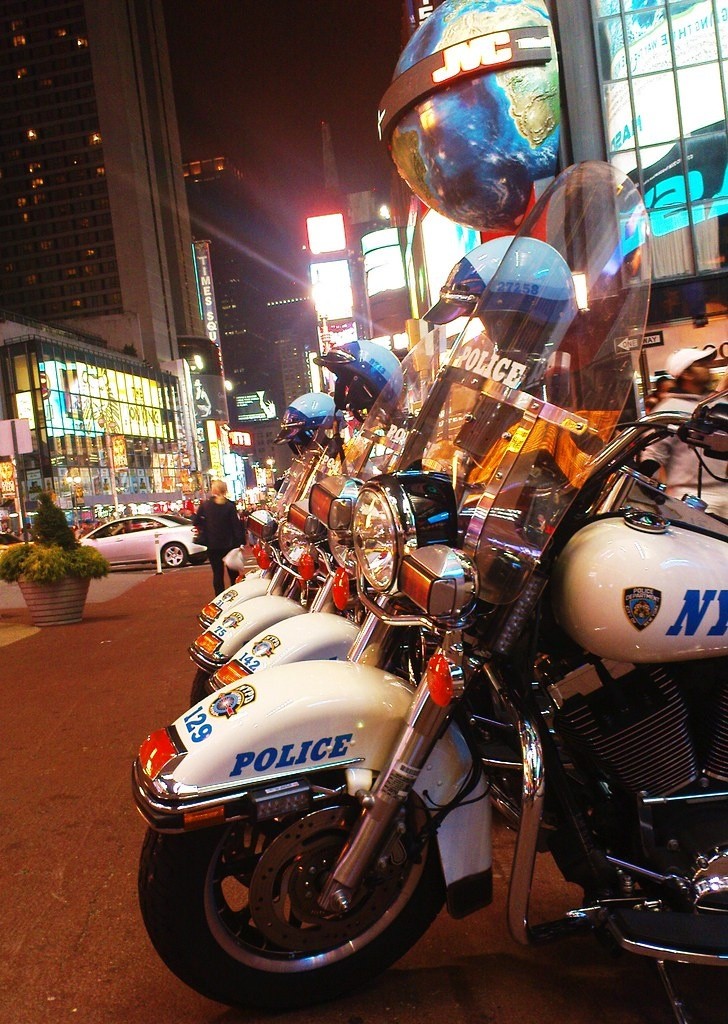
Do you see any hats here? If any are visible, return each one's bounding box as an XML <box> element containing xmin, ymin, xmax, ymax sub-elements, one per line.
<box><xmin>665</xmin><ymin>347</ymin><xmax>717</xmax><ymax>379</ymax></box>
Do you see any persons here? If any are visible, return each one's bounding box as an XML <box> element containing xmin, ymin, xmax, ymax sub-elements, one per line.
<box><xmin>184</xmin><ymin>508</ymin><xmax>195</xmax><ymax>520</ymax></box>
<box><xmin>644</xmin><ymin>346</ymin><xmax>728</xmax><ymax>520</ymax></box>
<box><xmin>193</xmin><ymin>379</ymin><xmax>212</xmax><ymax>418</ymax></box>
<box><xmin>29</xmin><ymin>481</ymin><xmax>42</xmax><ymax>493</ymax></box>
<box><xmin>654</xmin><ymin>376</ymin><xmax>675</xmax><ymax>393</ymax></box>
<box><xmin>191</xmin><ymin>478</ymin><xmax>247</xmax><ymax>597</ymax></box>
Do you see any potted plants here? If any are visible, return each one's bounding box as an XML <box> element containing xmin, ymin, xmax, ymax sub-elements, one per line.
<box><xmin>0</xmin><ymin>492</ymin><xmax>111</xmax><ymax>628</ymax></box>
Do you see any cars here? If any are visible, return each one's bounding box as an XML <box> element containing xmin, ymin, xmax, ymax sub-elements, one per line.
<box><xmin>80</xmin><ymin>516</ymin><xmax>208</xmax><ymax>569</ymax></box>
<box><xmin>0</xmin><ymin>532</ymin><xmax>35</xmax><ymax>564</ymax></box>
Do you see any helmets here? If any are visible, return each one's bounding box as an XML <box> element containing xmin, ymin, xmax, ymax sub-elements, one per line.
<box><xmin>422</xmin><ymin>236</ymin><xmax>578</xmax><ymax>337</ymax></box>
<box><xmin>277</xmin><ymin>392</ymin><xmax>345</xmax><ymax>438</ymax></box>
<box><xmin>274</xmin><ymin>429</ymin><xmax>316</xmax><ymax>454</ymax></box>
<box><xmin>312</xmin><ymin>340</ymin><xmax>403</xmax><ymax>415</ymax></box>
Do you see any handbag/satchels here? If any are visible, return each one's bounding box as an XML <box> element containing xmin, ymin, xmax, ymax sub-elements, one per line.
<box><xmin>192</xmin><ymin>502</ymin><xmax>208</xmax><ymax>546</ymax></box>
<box><xmin>222</xmin><ymin>545</ymin><xmax>245</xmax><ymax>571</ymax></box>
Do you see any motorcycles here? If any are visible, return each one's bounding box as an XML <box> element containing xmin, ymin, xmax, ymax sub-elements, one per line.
<box><xmin>128</xmin><ymin>156</ymin><xmax>728</xmax><ymax>1024</ymax></box>
